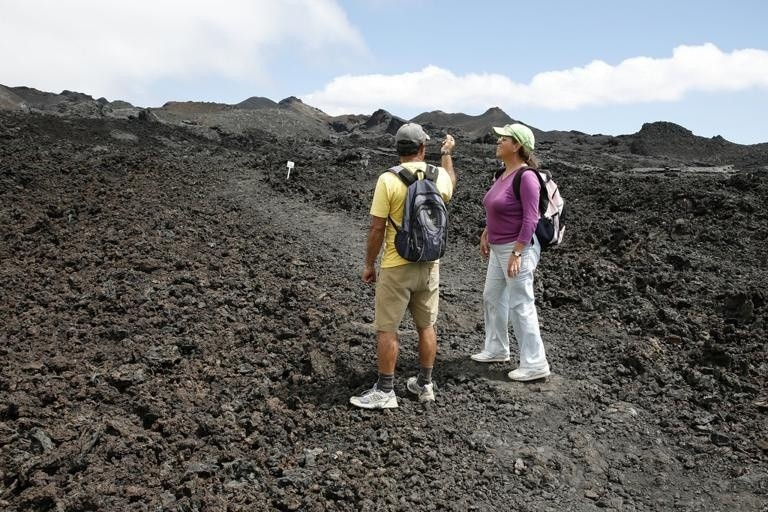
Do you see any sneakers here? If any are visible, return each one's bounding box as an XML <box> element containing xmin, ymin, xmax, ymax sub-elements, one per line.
<box><xmin>508</xmin><ymin>365</ymin><xmax>551</xmax><ymax>381</ymax></box>
<box><xmin>407</xmin><ymin>377</ymin><xmax>435</xmax><ymax>403</ymax></box>
<box><xmin>349</xmin><ymin>383</ymin><xmax>399</xmax><ymax>409</ymax></box>
<box><xmin>471</xmin><ymin>350</ymin><xmax>510</xmax><ymax>362</ymax></box>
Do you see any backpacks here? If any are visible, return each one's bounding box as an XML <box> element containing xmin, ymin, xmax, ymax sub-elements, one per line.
<box><xmin>382</xmin><ymin>164</ymin><xmax>448</xmax><ymax>263</ymax></box>
<box><xmin>495</xmin><ymin>167</ymin><xmax>565</xmax><ymax>247</ymax></box>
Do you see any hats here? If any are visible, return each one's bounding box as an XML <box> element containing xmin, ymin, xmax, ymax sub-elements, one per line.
<box><xmin>492</xmin><ymin>124</ymin><xmax>535</xmax><ymax>152</ymax></box>
<box><xmin>394</xmin><ymin>123</ymin><xmax>431</xmax><ymax>148</ymax></box>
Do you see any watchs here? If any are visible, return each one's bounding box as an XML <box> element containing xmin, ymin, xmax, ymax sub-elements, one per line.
<box><xmin>510</xmin><ymin>250</ymin><xmax>522</xmax><ymax>258</ymax></box>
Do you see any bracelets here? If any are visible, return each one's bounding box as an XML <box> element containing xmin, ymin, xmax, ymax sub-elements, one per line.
<box><xmin>440</xmin><ymin>150</ymin><xmax>452</xmax><ymax>157</ymax></box>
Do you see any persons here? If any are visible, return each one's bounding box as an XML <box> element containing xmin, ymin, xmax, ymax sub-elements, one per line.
<box><xmin>468</xmin><ymin>123</ymin><xmax>550</xmax><ymax>381</ymax></box>
<box><xmin>349</xmin><ymin>121</ymin><xmax>456</xmax><ymax>409</ymax></box>
